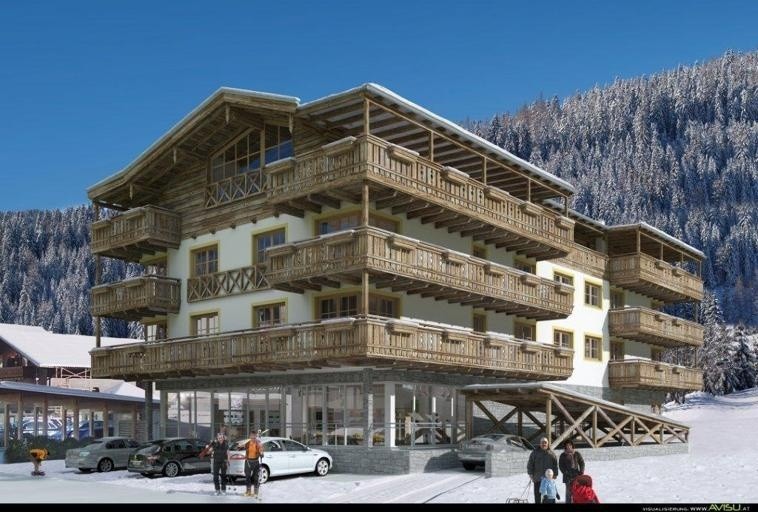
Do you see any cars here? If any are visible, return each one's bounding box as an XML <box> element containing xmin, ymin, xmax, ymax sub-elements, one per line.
<box><xmin>456</xmin><ymin>434</ymin><xmax>535</xmax><ymax>471</ymax></box>
<box><xmin>128</xmin><ymin>438</ymin><xmax>213</xmax><ymax>477</ymax></box>
<box><xmin>211</xmin><ymin>436</ymin><xmax>333</xmax><ymax>483</ymax></box>
<box><xmin>65</xmin><ymin>436</ymin><xmax>140</xmax><ymax>472</ymax></box>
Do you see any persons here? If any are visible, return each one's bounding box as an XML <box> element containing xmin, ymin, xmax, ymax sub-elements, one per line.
<box><xmin>570</xmin><ymin>474</ymin><xmax>598</xmax><ymax>504</ymax></box>
<box><xmin>241</xmin><ymin>430</ymin><xmax>264</xmax><ymax>496</ymax></box>
<box><xmin>205</xmin><ymin>432</ymin><xmax>231</xmax><ymax>495</ymax></box>
<box><xmin>526</xmin><ymin>436</ymin><xmax>558</xmax><ymax>503</ymax></box>
<box><xmin>538</xmin><ymin>469</ymin><xmax>560</xmax><ymax>503</ymax></box>
<box><xmin>558</xmin><ymin>439</ymin><xmax>585</xmax><ymax>503</ymax></box>
<box><xmin>29</xmin><ymin>447</ymin><xmax>50</xmax><ymax>473</ymax></box>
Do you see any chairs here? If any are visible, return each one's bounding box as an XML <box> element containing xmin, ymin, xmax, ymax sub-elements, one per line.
<box><xmin>165</xmin><ymin>444</ymin><xmax>204</xmax><ymax>451</ymax></box>
